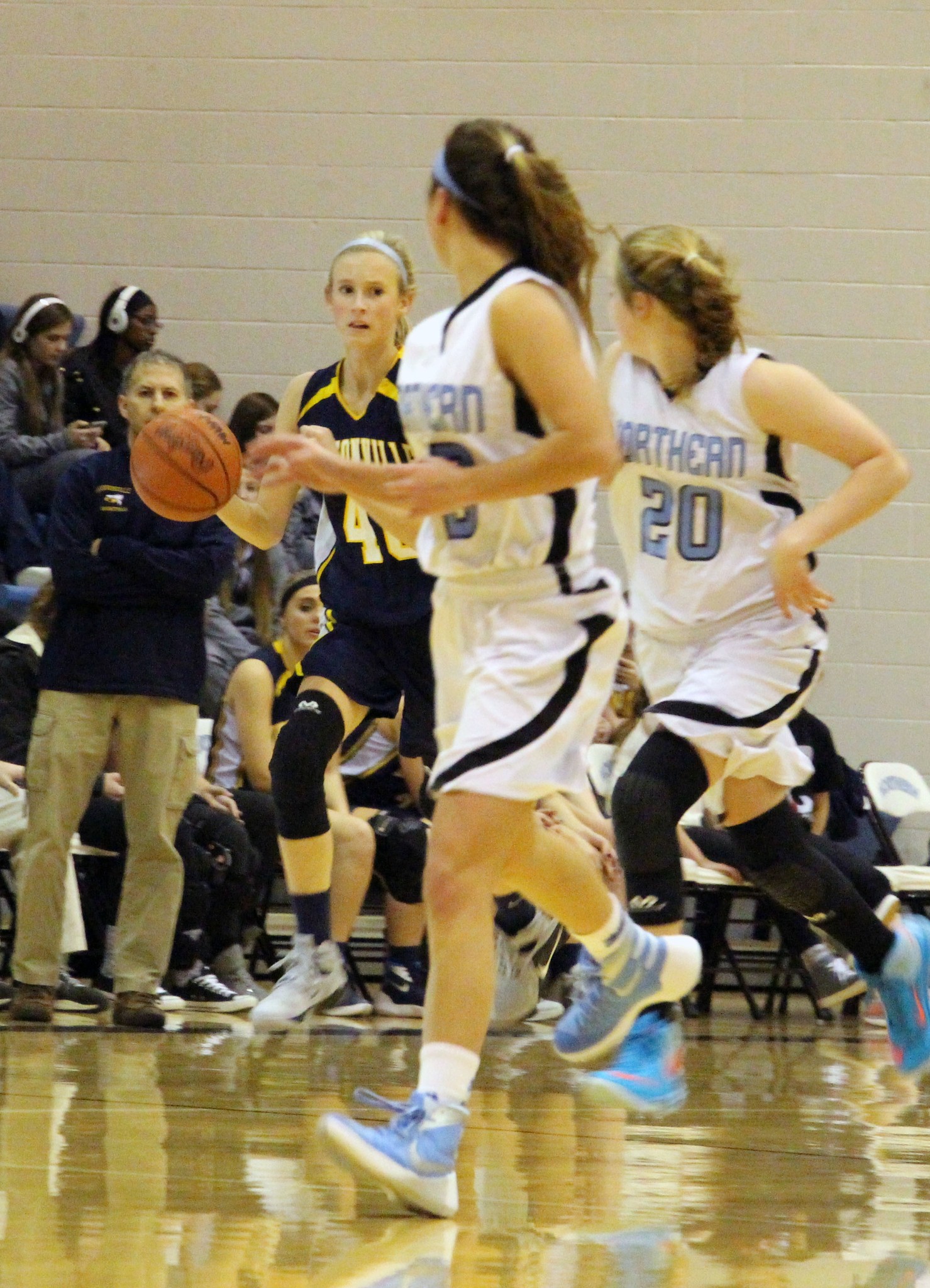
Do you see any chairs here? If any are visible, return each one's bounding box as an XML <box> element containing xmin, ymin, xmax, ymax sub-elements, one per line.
<box><xmin>266</xmin><ymin>740</ymin><xmax>930</xmax><ymax>1019</ymax></box>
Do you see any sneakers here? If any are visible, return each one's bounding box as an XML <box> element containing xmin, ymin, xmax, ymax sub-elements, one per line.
<box><xmin>252</xmin><ymin>942</ymin><xmax>348</xmax><ymax>1033</ymax></box>
<box><xmin>215</xmin><ymin>955</ymin><xmax>274</xmax><ymax>1002</ymax></box>
<box><xmin>489</xmin><ymin>910</ymin><xmax>560</xmax><ymax>1026</ymax></box>
<box><xmin>54</xmin><ymin>967</ymin><xmax>108</xmax><ymax>1013</ymax></box>
<box><xmin>857</xmin><ymin>913</ymin><xmax>929</xmax><ymax>1074</ymax></box>
<box><xmin>152</xmin><ymin>986</ymin><xmax>186</xmax><ymax>1008</ymax></box>
<box><xmin>863</xmin><ymin>1250</ymin><xmax>927</xmax><ymax>1288</ymax></box>
<box><xmin>168</xmin><ymin>1010</ymin><xmax>253</xmax><ymax>1068</ymax></box>
<box><xmin>328</xmin><ymin>1219</ymin><xmax>457</xmax><ymax>1288</ymax></box>
<box><xmin>321</xmin><ymin>1088</ymin><xmax>472</xmax><ymax>1219</ymax></box>
<box><xmin>11</xmin><ymin>981</ymin><xmax>54</xmax><ymax>1023</ymax></box>
<box><xmin>375</xmin><ymin>962</ymin><xmax>425</xmax><ymax>1015</ymax></box>
<box><xmin>580</xmin><ymin>1013</ymin><xmax>688</xmax><ymax>1115</ymax></box>
<box><xmin>485</xmin><ymin>1031</ymin><xmax>559</xmax><ymax>1089</ymax></box>
<box><xmin>172</xmin><ymin>962</ymin><xmax>257</xmax><ymax>1013</ymax></box>
<box><xmin>315</xmin><ymin>965</ymin><xmax>372</xmax><ymax>1018</ymax></box>
<box><xmin>0</xmin><ymin>980</ymin><xmax>12</xmax><ymax>1006</ymax></box>
<box><xmin>112</xmin><ymin>993</ymin><xmax>165</xmax><ymax>1029</ymax></box>
<box><xmin>811</xmin><ymin>954</ymin><xmax>868</xmax><ymax>1009</ymax></box>
<box><xmin>244</xmin><ymin>1156</ymin><xmax>316</xmax><ymax>1223</ymax></box>
<box><xmin>543</xmin><ymin>1232</ymin><xmax>677</xmax><ymax>1288</ymax></box>
<box><xmin>554</xmin><ymin>917</ymin><xmax>701</xmax><ymax>1062</ymax></box>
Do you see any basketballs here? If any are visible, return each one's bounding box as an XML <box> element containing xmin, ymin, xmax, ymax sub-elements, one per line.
<box><xmin>128</xmin><ymin>407</ymin><xmax>243</xmax><ymax>522</ymax></box>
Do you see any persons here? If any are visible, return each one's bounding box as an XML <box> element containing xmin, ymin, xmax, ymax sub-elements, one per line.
<box><xmin>0</xmin><ymin>282</ymin><xmax>930</xmax><ymax>1035</ymax></box>
<box><xmin>239</xmin><ymin>117</ymin><xmax>703</xmax><ymax>1222</ymax></box>
<box><xmin>141</xmin><ymin>228</ymin><xmax>569</xmax><ymax>1035</ymax></box>
<box><xmin>567</xmin><ymin>218</ymin><xmax>930</xmax><ymax>1126</ymax></box>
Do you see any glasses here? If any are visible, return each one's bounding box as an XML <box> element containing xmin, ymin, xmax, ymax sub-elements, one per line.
<box><xmin>138</xmin><ymin>315</ymin><xmax>163</xmax><ymax>330</ymax></box>
